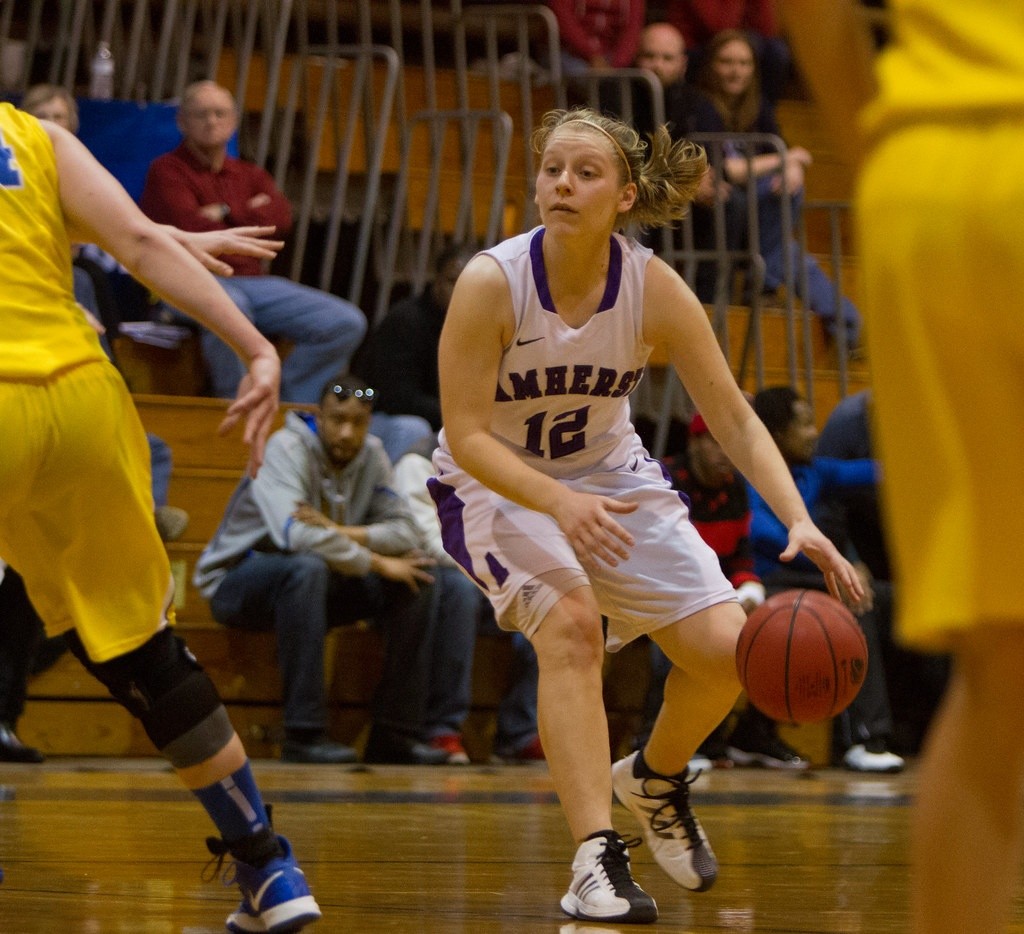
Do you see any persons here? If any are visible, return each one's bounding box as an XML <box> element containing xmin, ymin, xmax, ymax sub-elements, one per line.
<box><xmin>20</xmin><ymin>88</ymin><xmax>133</xmax><ymax>362</ymax></box>
<box><xmin>0</xmin><ymin>223</ymin><xmax>285</xmax><ymax>800</ymax></box>
<box><xmin>535</xmin><ymin>1</ymin><xmax>784</xmax><ymax>109</ymax></box>
<box><xmin>629</xmin><ymin>384</ymin><xmax>954</xmax><ymax>775</ymax></box>
<box><xmin>392</xmin><ymin>432</ymin><xmax>548</xmax><ymax>766</ymax></box>
<box><xmin>685</xmin><ymin>29</ymin><xmax>859</xmax><ymax>371</ymax></box>
<box><xmin>0</xmin><ymin>102</ymin><xmax>322</xmax><ymax>933</ymax></box>
<box><xmin>139</xmin><ymin>81</ymin><xmax>368</xmax><ymax>403</ymax></box>
<box><xmin>191</xmin><ymin>375</ymin><xmax>451</xmax><ymax>765</ymax></box>
<box><xmin>348</xmin><ymin>242</ymin><xmax>483</xmax><ymax>466</ymax></box>
<box><xmin>425</xmin><ymin>109</ymin><xmax>864</xmax><ymax>924</ymax></box>
<box><xmin>785</xmin><ymin>0</ymin><xmax>1024</xmax><ymax>934</ymax></box>
<box><xmin>583</xmin><ymin>22</ymin><xmax>733</xmax><ymax>303</ymax></box>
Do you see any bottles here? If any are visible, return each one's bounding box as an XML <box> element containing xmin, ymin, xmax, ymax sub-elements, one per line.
<box><xmin>89</xmin><ymin>41</ymin><xmax>114</xmax><ymax>101</ymax></box>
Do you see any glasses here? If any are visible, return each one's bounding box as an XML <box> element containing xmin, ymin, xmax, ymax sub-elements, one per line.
<box><xmin>334</xmin><ymin>385</ymin><xmax>378</xmax><ymax>401</ymax></box>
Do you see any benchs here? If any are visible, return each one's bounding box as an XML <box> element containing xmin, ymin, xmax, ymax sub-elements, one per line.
<box><xmin>0</xmin><ymin>0</ymin><xmax>871</xmax><ymax>765</ymax></box>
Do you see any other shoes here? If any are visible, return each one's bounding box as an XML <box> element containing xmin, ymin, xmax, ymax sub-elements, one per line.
<box><xmin>365</xmin><ymin>738</ymin><xmax>447</xmax><ymax>766</ymax></box>
<box><xmin>0</xmin><ymin>726</ymin><xmax>44</xmax><ymax>763</ymax></box>
<box><xmin>279</xmin><ymin>734</ymin><xmax>357</xmax><ymax>763</ymax></box>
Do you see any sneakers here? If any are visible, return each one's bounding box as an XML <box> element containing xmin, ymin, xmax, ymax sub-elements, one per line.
<box><xmin>612</xmin><ymin>749</ymin><xmax>718</xmax><ymax>892</ymax></box>
<box><xmin>838</xmin><ymin>741</ymin><xmax>904</xmax><ymax>774</ymax></box>
<box><xmin>559</xmin><ymin>831</ymin><xmax>660</xmax><ymax>924</ymax></box>
<box><xmin>226</xmin><ymin>835</ymin><xmax>325</xmax><ymax>932</ymax></box>
<box><xmin>630</xmin><ymin>738</ymin><xmax>712</xmax><ymax>773</ymax></box>
<box><xmin>430</xmin><ymin>735</ymin><xmax>470</xmax><ymax>764</ymax></box>
<box><xmin>727</xmin><ymin>728</ymin><xmax>810</xmax><ymax>770</ymax></box>
<box><xmin>485</xmin><ymin>734</ymin><xmax>560</xmax><ymax>767</ymax></box>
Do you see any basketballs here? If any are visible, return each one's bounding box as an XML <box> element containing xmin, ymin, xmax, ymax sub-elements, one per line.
<box><xmin>736</xmin><ymin>589</ymin><xmax>868</xmax><ymax>728</ymax></box>
